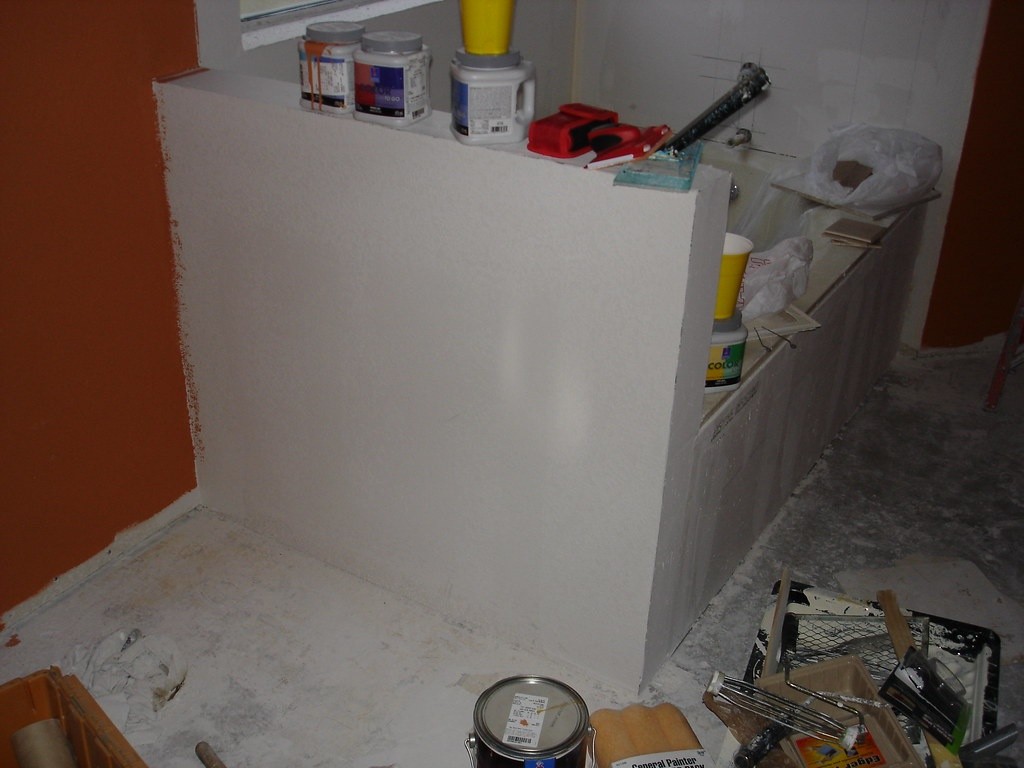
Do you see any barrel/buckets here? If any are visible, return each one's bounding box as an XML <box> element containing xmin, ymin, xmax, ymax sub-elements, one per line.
<box><xmin>464</xmin><ymin>674</ymin><xmax>595</xmax><ymax>768</ymax></box>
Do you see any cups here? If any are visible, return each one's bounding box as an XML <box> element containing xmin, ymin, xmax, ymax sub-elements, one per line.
<box><xmin>459</xmin><ymin>0</ymin><xmax>515</xmax><ymax>54</ymax></box>
<box><xmin>714</xmin><ymin>233</ymin><xmax>754</xmax><ymax>319</ymax></box>
